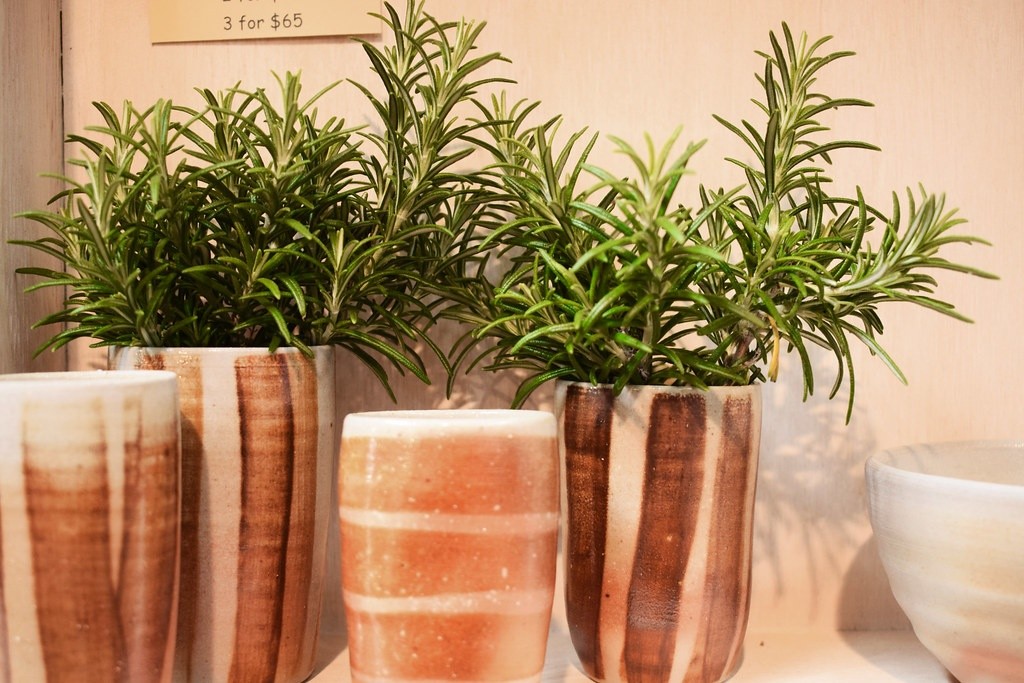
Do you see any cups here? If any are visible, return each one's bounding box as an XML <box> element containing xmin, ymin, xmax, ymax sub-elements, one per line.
<box><xmin>339</xmin><ymin>409</ymin><xmax>562</xmax><ymax>683</ymax></box>
<box><xmin>0</xmin><ymin>370</ymin><xmax>181</xmax><ymax>683</ymax></box>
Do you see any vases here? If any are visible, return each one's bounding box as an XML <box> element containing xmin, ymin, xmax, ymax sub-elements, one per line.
<box><xmin>0</xmin><ymin>369</ymin><xmax>184</xmax><ymax>683</ymax></box>
<box><xmin>861</xmin><ymin>440</ymin><xmax>1024</xmax><ymax>683</ymax></box>
<box><xmin>335</xmin><ymin>412</ymin><xmax>561</xmax><ymax>683</ymax></box>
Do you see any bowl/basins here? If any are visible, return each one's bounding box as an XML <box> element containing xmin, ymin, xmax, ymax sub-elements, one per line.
<box><xmin>864</xmin><ymin>442</ymin><xmax>1024</xmax><ymax>683</ymax></box>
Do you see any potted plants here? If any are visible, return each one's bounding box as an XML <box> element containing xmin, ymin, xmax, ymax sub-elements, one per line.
<box><xmin>416</xmin><ymin>22</ymin><xmax>1003</xmax><ymax>683</ymax></box>
<box><xmin>8</xmin><ymin>0</ymin><xmax>517</xmax><ymax>683</ymax></box>
